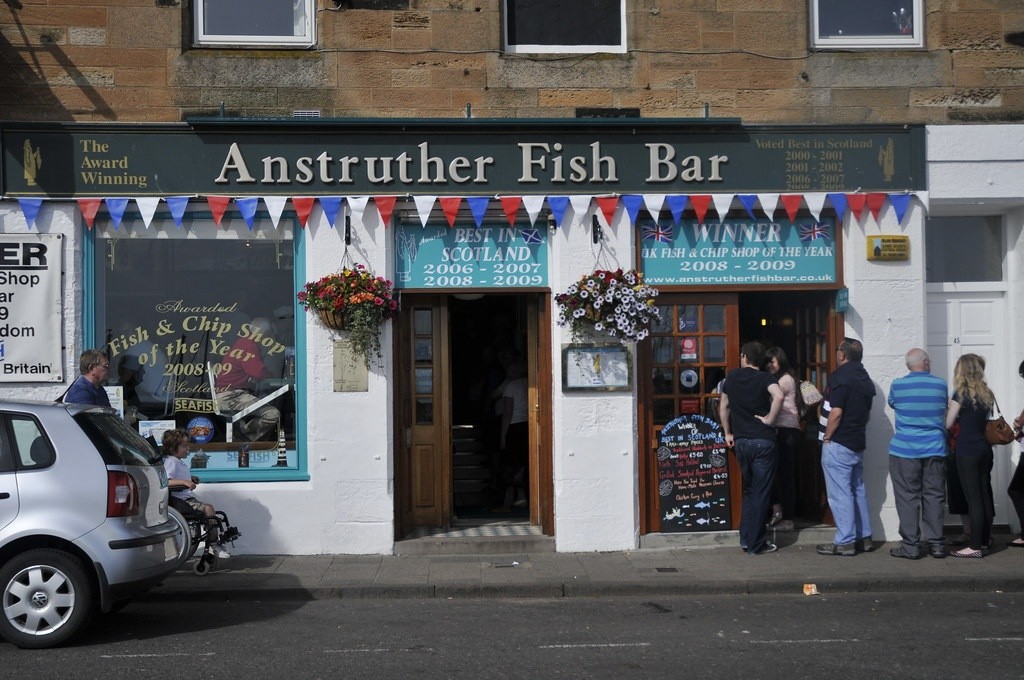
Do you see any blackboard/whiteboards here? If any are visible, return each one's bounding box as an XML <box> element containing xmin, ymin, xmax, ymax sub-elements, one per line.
<box><xmin>657</xmin><ymin>414</ymin><xmax>732</xmax><ymax>533</ymax></box>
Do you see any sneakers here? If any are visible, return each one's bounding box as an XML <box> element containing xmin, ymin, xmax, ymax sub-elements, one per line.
<box><xmin>931</xmin><ymin>545</ymin><xmax>945</xmax><ymax>558</ymax></box>
<box><xmin>208</xmin><ymin>546</ymin><xmax>230</xmax><ymax>558</ymax></box>
<box><xmin>891</xmin><ymin>544</ymin><xmax>919</xmax><ymax>559</ymax></box>
<box><xmin>816</xmin><ymin>544</ymin><xmax>855</xmax><ymax>555</ymax></box>
<box><xmin>855</xmin><ymin>536</ymin><xmax>873</xmax><ymax>552</ymax></box>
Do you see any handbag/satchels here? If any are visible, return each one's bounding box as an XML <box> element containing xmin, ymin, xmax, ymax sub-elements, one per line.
<box><xmin>983</xmin><ymin>417</ymin><xmax>1015</xmax><ymax>445</ymax></box>
<box><xmin>800</xmin><ymin>380</ymin><xmax>823</xmax><ymax>407</ymax></box>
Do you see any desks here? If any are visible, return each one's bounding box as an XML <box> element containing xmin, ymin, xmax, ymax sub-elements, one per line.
<box><xmin>257</xmin><ymin>378</ymin><xmax>295</xmax><ymax>390</ymax></box>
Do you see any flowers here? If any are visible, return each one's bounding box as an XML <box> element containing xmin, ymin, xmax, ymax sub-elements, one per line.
<box><xmin>296</xmin><ymin>263</ymin><xmax>397</xmax><ymax>379</ymax></box>
<box><xmin>554</xmin><ymin>269</ymin><xmax>663</xmax><ymax>347</ymax></box>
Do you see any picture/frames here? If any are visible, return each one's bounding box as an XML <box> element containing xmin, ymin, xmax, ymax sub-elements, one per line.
<box><xmin>560</xmin><ymin>343</ymin><xmax>633</xmax><ymax>392</ymax></box>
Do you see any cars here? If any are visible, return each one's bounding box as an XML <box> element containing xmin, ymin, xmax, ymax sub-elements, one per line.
<box><xmin>0</xmin><ymin>400</ymin><xmax>191</xmax><ymax>651</ymax></box>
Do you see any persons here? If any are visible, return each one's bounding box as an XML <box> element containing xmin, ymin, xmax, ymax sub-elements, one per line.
<box><xmin>945</xmin><ymin>354</ymin><xmax>996</xmax><ymax>558</ymax></box>
<box><xmin>115</xmin><ymin>355</ymin><xmax>146</xmax><ymax>414</ymax></box>
<box><xmin>764</xmin><ymin>347</ymin><xmax>801</xmax><ymax>532</ymax></box>
<box><xmin>65</xmin><ymin>349</ymin><xmax>112</xmax><ymax>407</ymax></box>
<box><xmin>118</xmin><ymin>318</ymin><xmax>180</xmax><ymax>403</ymax></box>
<box><xmin>161</xmin><ymin>429</ymin><xmax>231</xmax><ymax>558</ymax></box>
<box><xmin>216</xmin><ymin>306</ymin><xmax>294</xmax><ymax>442</ymax></box>
<box><xmin>706</xmin><ymin>359</ymin><xmax>725</xmax><ymax>423</ymax></box>
<box><xmin>1007</xmin><ymin>361</ymin><xmax>1024</xmax><ymax>547</ymax></box>
<box><xmin>719</xmin><ymin>342</ymin><xmax>784</xmax><ymax>555</ymax></box>
<box><xmin>474</xmin><ymin>345</ymin><xmax>528</xmax><ymax>513</ymax></box>
<box><xmin>815</xmin><ymin>338</ymin><xmax>877</xmax><ymax>556</ymax></box>
<box><xmin>888</xmin><ymin>348</ymin><xmax>949</xmax><ymax>560</ymax></box>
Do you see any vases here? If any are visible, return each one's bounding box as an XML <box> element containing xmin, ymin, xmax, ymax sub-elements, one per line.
<box><xmin>316</xmin><ymin>307</ymin><xmax>350</xmax><ymax>330</ymax></box>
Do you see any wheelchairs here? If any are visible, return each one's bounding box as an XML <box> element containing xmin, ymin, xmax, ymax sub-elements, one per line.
<box><xmin>140</xmin><ymin>434</ymin><xmax>242</xmax><ymax>576</ymax></box>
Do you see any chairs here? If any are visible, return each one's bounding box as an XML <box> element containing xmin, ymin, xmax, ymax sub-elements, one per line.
<box><xmin>151</xmin><ymin>371</ymin><xmax>178</xmax><ymax>419</ymax></box>
<box><xmin>30</xmin><ymin>436</ymin><xmax>51</xmax><ymax>464</ymax></box>
<box><xmin>207</xmin><ymin>361</ymin><xmax>235</xmax><ymax>442</ymax></box>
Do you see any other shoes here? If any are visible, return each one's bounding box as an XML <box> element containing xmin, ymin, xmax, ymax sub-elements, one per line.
<box><xmin>949</xmin><ymin>550</ymin><xmax>982</xmax><ymax>558</ymax></box>
<box><xmin>981</xmin><ymin>536</ymin><xmax>993</xmax><ymax>556</ymax></box>
<box><xmin>769</xmin><ymin>511</ymin><xmax>782</xmax><ymax>526</ymax></box>
<box><xmin>751</xmin><ymin>543</ymin><xmax>777</xmax><ymax>554</ymax></box>
<box><xmin>1006</xmin><ymin>539</ymin><xmax>1024</xmax><ymax>548</ymax></box>
<box><xmin>948</xmin><ymin>540</ymin><xmax>972</xmax><ymax>546</ymax></box>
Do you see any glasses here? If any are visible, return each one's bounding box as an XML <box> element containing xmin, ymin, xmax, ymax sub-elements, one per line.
<box><xmin>94</xmin><ymin>363</ymin><xmax>110</xmax><ymax>369</ymax></box>
